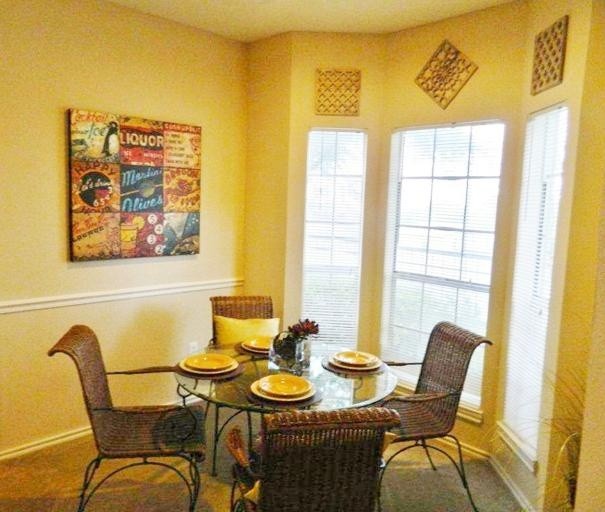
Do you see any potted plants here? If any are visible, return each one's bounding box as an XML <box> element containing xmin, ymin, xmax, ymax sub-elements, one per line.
<box><xmin>487</xmin><ymin>366</ymin><xmax>586</xmax><ymax>511</ymax></box>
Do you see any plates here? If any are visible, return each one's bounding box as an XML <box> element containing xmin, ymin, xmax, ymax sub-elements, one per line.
<box><xmin>184</xmin><ymin>353</ymin><xmax>236</xmax><ymax>371</ymax></box>
<box><xmin>333</xmin><ymin>349</ymin><xmax>378</xmax><ymax>366</ymax></box>
<box><xmin>178</xmin><ymin>360</ymin><xmax>239</xmax><ymax>375</ymax></box>
<box><xmin>328</xmin><ymin>357</ymin><xmax>382</xmax><ymax>371</ymax></box>
<box><xmin>245</xmin><ymin>335</ymin><xmax>274</xmax><ymax>350</ymax></box>
<box><xmin>242</xmin><ymin>342</ymin><xmax>270</xmax><ymax>354</ymax></box>
<box><xmin>249</xmin><ymin>379</ymin><xmax>316</xmax><ymax>402</ymax></box>
<box><xmin>258</xmin><ymin>374</ymin><xmax>312</xmax><ymax>396</ymax></box>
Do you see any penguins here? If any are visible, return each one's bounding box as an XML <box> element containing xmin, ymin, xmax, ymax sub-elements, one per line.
<box><xmin>101</xmin><ymin>121</ymin><xmax>119</xmax><ymax>156</ymax></box>
